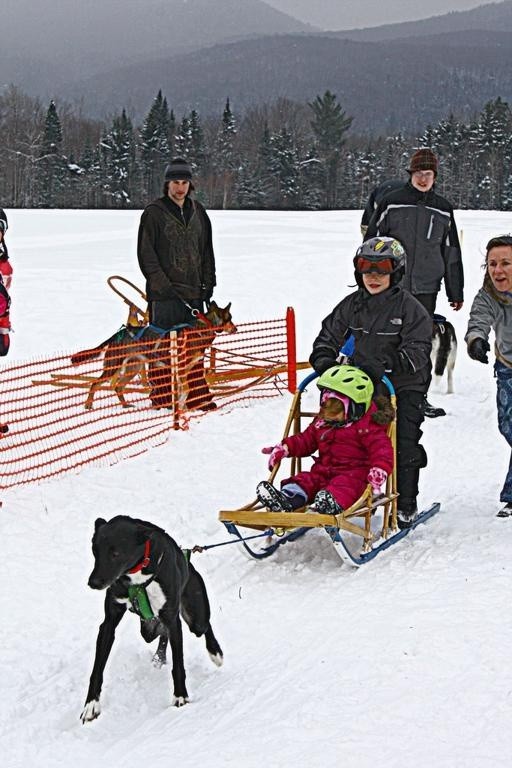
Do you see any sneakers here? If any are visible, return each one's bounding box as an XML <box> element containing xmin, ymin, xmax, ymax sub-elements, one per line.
<box><xmin>496</xmin><ymin>503</ymin><xmax>512</xmax><ymax>521</ymax></box>
<box><xmin>387</xmin><ymin>510</ymin><xmax>419</xmax><ymax>528</ymax></box>
<box><xmin>152</xmin><ymin>400</ymin><xmax>178</xmax><ymax>409</ymax></box>
<box><xmin>418</xmin><ymin>395</ymin><xmax>446</xmax><ymax>417</ymax></box>
<box><xmin>184</xmin><ymin>398</ymin><xmax>217</xmax><ymax>411</ymax></box>
<box><xmin>256</xmin><ymin>478</ymin><xmax>293</xmax><ymax>517</ymax></box>
<box><xmin>304</xmin><ymin>490</ymin><xmax>342</xmax><ymax>519</ymax></box>
<box><xmin>0</xmin><ymin>421</ymin><xmax>8</xmax><ymax>432</ymax></box>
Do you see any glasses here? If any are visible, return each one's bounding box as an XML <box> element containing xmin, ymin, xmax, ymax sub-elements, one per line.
<box><xmin>356</xmin><ymin>257</ymin><xmax>396</xmax><ymax>274</ymax></box>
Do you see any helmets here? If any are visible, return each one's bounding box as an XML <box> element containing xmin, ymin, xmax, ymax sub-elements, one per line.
<box><xmin>353</xmin><ymin>236</ymin><xmax>408</xmax><ymax>276</ymax></box>
<box><xmin>317</xmin><ymin>365</ymin><xmax>374</xmax><ymax>413</ymax></box>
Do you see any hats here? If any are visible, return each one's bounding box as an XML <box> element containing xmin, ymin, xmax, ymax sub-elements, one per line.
<box><xmin>164</xmin><ymin>159</ymin><xmax>193</xmax><ymax>185</ymax></box>
<box><xmin>404</xmin><ymin>148</ymin><xmax>438</xmax><ymax>175</ymax></box>
<box><xmin>0</xmin><ymin>209</ymin><xmax>9</xmax><ymax>233</ymax></box>
<box><xmin>320</xmin><ymin>388</ymin><xmax>351</xmax><ymax>421</ymax></box>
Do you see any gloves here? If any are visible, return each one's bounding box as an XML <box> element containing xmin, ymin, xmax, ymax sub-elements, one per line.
<box><xmin>0</xmin><ymin>328</ymin><xmax>11</xmax><ymax>355</ymax></box>
<box><xmin>262</xmin><ymin>446</ymin><xmax>289</xmax><ymax>472</ymax></box>
<box><xmin>470</xmin><ymin>339</ymin><xmax>490</xmax><ymax>365</ymax></box>
<box><xmin>203</xmin><ymin>288</ymin><xmax>213</xmax><ymax>302</ymax></box>
<box><xmin>157</xmin><ymin>282</ymin><xmax>177</xmax><ymax>298</ymax></box>
<box><xmin>367</xmin><ymin>467</ymin><xmax>388</xmax><ymax>489</ymax></box>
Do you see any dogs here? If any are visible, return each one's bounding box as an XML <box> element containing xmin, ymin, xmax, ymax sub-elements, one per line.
<box><xmin>429</xmin><ymin>320</ymin><xmax>457</xmax><ymax>394</ymax></box>
<box><xmin>80</xmin><ymin>515</ymin><xmax>223</xmax><ymax>724</ymax></box>
<box><xmin>71</xmin><ymin>302</ymin><xmax>238</xmax><ymax>412</ymax></box>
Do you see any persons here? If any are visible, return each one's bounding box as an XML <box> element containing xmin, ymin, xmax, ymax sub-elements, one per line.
<box><xmin>257</xmin><ymin>364</ymin><xmax>395</xmax><ymax>516</ymax></box>
<box><xmin>463</xmin><ymin>235</ymin><xmax>512</xmax><ymax>518</ymax></box>
<box><xmin>310</xmin><ymin>236</ymin><xmax>436</xmax><ymax>529</ymax></box>
<box><xmin>0</xmin><ymin>207</ymin><xmax>17</xmax><ymax>357</ymax></box>
<box><xmin>135</xmin><ymin>158</ymin><xmax>217</xmax><ymax>411</ymax></box>
<box><xmin>361</xmin><ymin>149</ymin><xmax>464</xmax><ymax>320</ymax></box>
